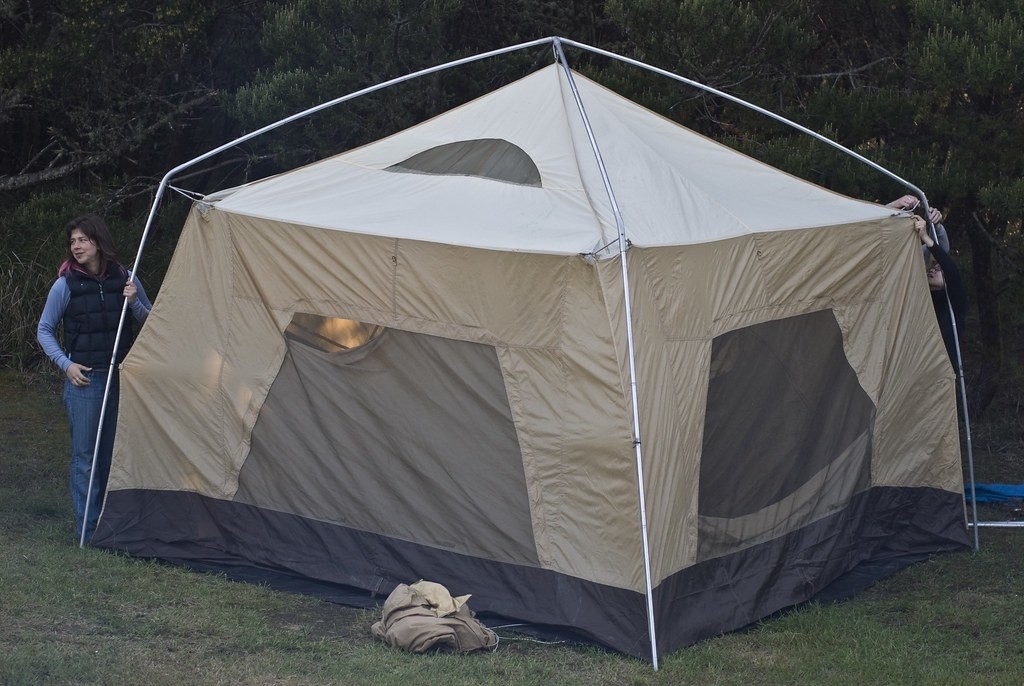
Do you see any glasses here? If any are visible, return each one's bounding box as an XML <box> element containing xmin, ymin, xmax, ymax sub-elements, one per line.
<box><xmin>936</xmin><ymin>266</ymin><xmax>941</xmax><ymax>270</ymax></box>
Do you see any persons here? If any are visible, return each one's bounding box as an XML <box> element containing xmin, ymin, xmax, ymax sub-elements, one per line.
<box><xmin>36</xmin><ymin>213</ymin><xmax>153</xmax><ymax>544</ymax></box>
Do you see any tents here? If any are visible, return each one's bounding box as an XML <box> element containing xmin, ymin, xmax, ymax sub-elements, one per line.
<box><xmin>80</xmin><ymin>37</ymin><xmax>985</xmax><ymax>668</ymax></box>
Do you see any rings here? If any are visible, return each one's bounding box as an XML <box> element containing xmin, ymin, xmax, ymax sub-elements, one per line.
<box><xmin>76</xmin><ymin>378</ymin><xmax>78</xmax><ymax>382</ymax></box>
